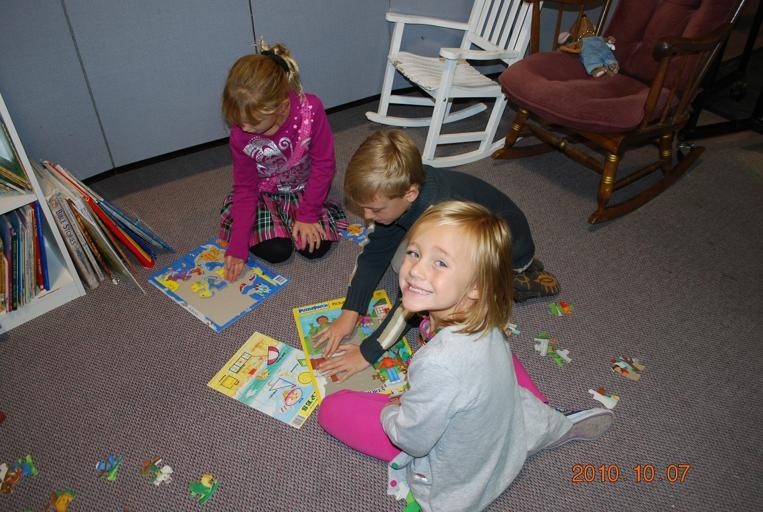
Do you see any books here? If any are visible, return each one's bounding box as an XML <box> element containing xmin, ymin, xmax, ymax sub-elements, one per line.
<box><xmin>0</xmin><ymin>120</ymin><xmax>176</xmax><ymax>316</ymax></box>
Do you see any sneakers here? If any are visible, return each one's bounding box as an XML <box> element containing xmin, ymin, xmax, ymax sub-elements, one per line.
<box><xmin>542</xmin><ymin>404</ymin><xmax>615</xmax><ymax>451</ymax></box>
<box><xmin>511</xmin><ymin>257</ymin><xmax>561</xmax><ymax>304</ymax></box>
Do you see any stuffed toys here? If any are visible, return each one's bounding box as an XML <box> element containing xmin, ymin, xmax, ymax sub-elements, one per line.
<box><xmin>558</xmin><ymin>15</ymin><xmax>620</xmax><ymax>78</ymax></box>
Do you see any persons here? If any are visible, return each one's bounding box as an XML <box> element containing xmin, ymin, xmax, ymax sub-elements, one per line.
<box><xmin>312</xmin><ymin>131</ymin><xmax>562</xmax><ymax>384</ymax></box>
<box><xmin>222</xmin><ymin>45</ymin><xmax>349</xmax><ymax>283</ymax></box>
<box><xmin>319</xmin><ymin>201</ymin><xmax>615</xmax><ymax>512</ymax></box>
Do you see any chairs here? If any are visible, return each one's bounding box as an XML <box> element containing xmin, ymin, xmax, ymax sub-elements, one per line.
<box><xmin>489</xmin><ymin>1</ymin><xmax>745</xmax><ymax>226</ymax></box>
<box><xmin>365</xmin><ymin>0</ymin><xmax>548</xmax><ymax>171</ymax></box>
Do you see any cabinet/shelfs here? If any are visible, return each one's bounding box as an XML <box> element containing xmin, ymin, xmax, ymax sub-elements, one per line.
<box><xmin>0</xmin><ymin>94</ymin><xmax>86</xmax><ymax>335</ymax></box>
